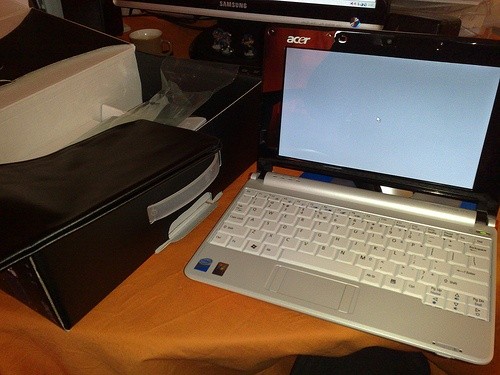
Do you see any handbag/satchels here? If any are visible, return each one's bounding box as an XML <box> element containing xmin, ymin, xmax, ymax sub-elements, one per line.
<box><xmin>0</xmin><ymin>7</ymin><xmax>143</xmax><ymax>166</ymax></box>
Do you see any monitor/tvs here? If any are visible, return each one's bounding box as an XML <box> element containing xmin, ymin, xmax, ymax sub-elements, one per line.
<box><xmin>111</xmin><ymin>0</ymin><xmax>392</xmax><ymax>31</ymax></box>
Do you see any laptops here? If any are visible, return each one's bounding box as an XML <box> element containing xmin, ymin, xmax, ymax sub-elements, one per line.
<box><xmin>185</xmin><ymin>30</ymin><xmax>500</xmax><ymax>363</ymax></box>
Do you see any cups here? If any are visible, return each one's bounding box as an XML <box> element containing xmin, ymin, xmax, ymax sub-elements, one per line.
<box><xmin>128</xmin><ymin>28</ymin><xmax>173</xmax><ymax>56</ymax></box>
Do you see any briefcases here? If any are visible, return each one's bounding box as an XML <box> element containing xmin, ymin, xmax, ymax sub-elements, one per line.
<box><xmin>0</xmin><ymin>51</ymin><xmax>270</xmax><ymax>332</ymax></box>
